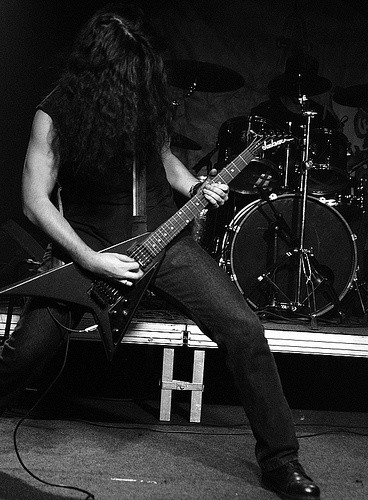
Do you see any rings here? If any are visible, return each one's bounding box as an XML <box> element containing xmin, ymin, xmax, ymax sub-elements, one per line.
<box><xmin>125</xmin><ymin>280</ymin><xmax>127</xmax><ymax>285</ymax></box>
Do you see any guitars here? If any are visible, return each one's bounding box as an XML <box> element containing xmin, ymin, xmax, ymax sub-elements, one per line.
<box><xmin>0</xmin><ymin>123</ymin><xmax>295</xmax><ymax>361</ymax></box>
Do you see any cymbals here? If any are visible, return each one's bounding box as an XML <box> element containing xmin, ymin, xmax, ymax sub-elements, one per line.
<box><xmin>269</xmin><ymin>72</ymin><xmax>332</xmax><ymax>96</ymax></box>
<box><xmin>169</xmin><ymin>131</ymin><xmax>202</xmax><ymax>150</ymax></box>
<box><xmin>333</xmin><ymin>83</ymin><xmax>368</xmax><ymax>107</ymax></box>
<box><xmin>167</xmin><ymin>58</ymin><xmax>245</xmax><ymax>92</ymax></box>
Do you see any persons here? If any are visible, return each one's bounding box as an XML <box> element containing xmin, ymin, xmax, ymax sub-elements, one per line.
<box><xmin>0</xmin><ymin>3</ymin><xmax>321</xmax><ymax>500</ymax></box>
<box><xmin>250</xmin><ymin>56</ymin><xmax>340</xmax><ymax>183</ymax></box>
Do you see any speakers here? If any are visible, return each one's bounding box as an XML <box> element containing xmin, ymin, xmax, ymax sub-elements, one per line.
<box><xmin>0</xmin><ymin>218</ymin><xmax>47</xmax><ymax>289</ymax></box>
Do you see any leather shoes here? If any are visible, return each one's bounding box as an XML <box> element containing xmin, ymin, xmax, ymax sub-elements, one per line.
<box><xmin>259</xmin><ymin>461</ymin><xmax>320</xmax><ymax>498</ymax></box>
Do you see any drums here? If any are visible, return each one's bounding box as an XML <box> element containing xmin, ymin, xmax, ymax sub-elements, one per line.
<box><xmin>216</xmin><ymin>114</ymin><xmax>288</xmax><ymax>195</ymax></box>
<box><xmin>289</xmin><ymin>127</ymin><xmax>349</xmax><ymax>196</ymax></box>
<box><xmin>220</xmin><ymin>192</ymin><xmax>358</xmax><ymax>322</ymax></box>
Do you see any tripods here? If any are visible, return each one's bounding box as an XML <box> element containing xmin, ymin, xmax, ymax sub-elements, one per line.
<box><xmin>253</xmin><ymin>79</ymin><xmax>346</xmax><ymax>331</ymax></box>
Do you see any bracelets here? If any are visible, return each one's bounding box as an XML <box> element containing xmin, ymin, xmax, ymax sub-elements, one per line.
<box><xmin>189</xmin><ymin>182</ymin><xmax>203</xmax><ymax>196</ymax></box>
